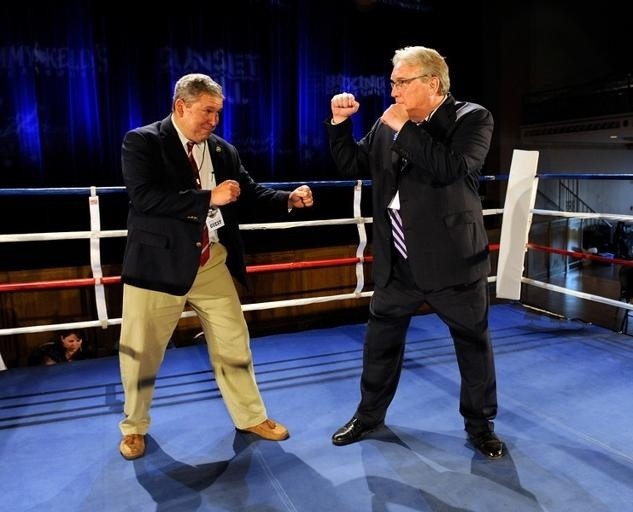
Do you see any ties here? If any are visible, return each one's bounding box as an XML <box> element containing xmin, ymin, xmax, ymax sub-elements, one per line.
<box><xmin>186</xmin><ymin>141</ymin><xmax>210</xmax><ymax>267</ymax></box>
<box><xmin>389</xmin><ymin>208</ymin><xmax>407</xmax><ymax>259</ymax></box>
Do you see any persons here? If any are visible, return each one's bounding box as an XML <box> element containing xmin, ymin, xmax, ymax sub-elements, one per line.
<box><xmin>323</xmin><ymin>47</ymin><xmax>508</xmax><ymax>460</ymax></box>
<box><xmin>619</xmin><ymin>265</ymin><xmax>633</xmax><ymax>304</ymax></box>
<box><xmin>25</xmin><ymin>320</ymin><xmax>96</xmax><ymax>367</ymax></box>
<box><xmin>120</xmin><ymin>73</ymin><xmax>314</xmax><ymax>458</ymax></box>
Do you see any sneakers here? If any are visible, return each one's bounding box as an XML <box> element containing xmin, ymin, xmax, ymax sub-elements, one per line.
<box><xmin>120</xmin><ymin>434</ymin><xmax>146</xmax><ymax>459</ymax></box>
<box><xmin>246</xmin><ymin>420</ymin><xmax>288</xmax><ymax>441</ymax></box>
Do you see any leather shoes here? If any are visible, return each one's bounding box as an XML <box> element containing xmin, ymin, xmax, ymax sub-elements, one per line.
<box><xmin>335</xmin><ymin>418</ymin><xmax>387</xmax><ymax>446</ymax></box>
<box><xmin>466</xmin><ymin>430</ymin><xmax>503</xmax><ymax>459</ymax></box>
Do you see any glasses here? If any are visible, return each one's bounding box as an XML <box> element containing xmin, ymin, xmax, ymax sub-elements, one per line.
<box><xmin>388</xmin><ymin>75</ymin><xmax>427</xmax><ymax>87</ymax></box>
